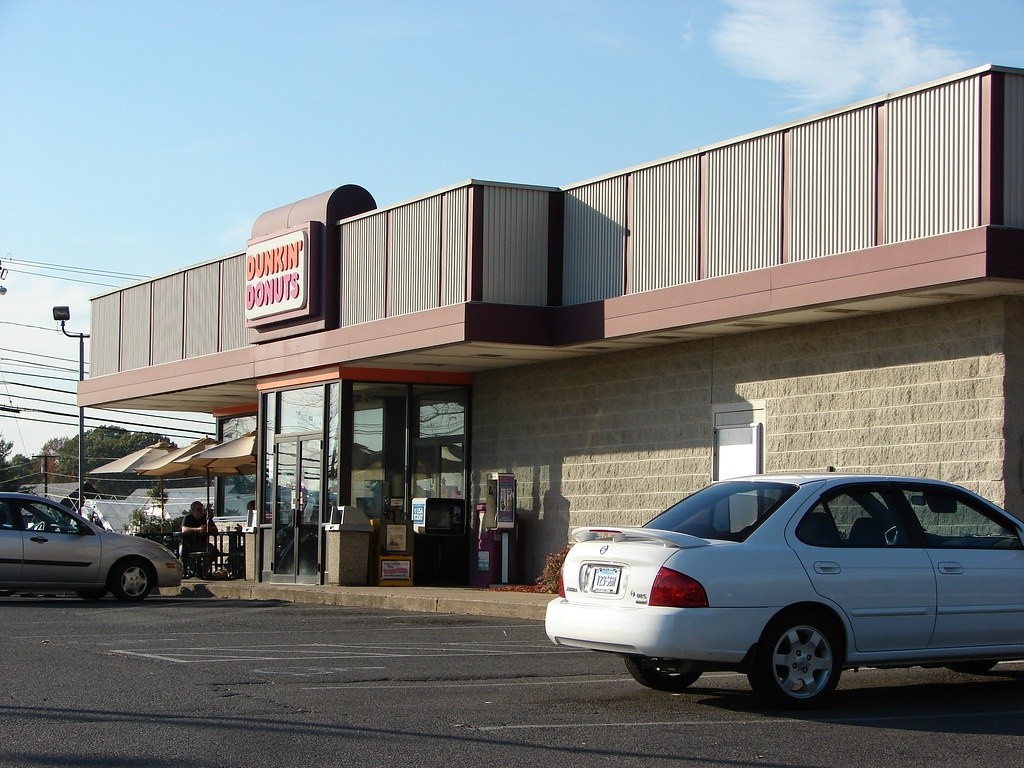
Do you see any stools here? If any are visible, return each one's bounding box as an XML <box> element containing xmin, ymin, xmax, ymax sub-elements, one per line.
<box><xmin>189</xmin><ymin>552</ymin><xmax>215</xmax><ymax>580</ymax></box>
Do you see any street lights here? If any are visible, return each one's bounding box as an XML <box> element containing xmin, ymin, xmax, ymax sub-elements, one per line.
<box><xmin>52</xmin><ymin>305</ymin><xmax>91</xmax><ymax>518</ymax></box>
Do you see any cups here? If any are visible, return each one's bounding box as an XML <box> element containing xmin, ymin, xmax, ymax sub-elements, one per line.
<box><xmin>225</xmin><ymin>526</ymin><xmax>230</xmax><ymax>532</ymax></box>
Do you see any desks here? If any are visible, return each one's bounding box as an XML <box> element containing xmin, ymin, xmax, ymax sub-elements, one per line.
<box><xmin>172</xmin><ymin>531</ymin><xmax>246</xmax><ymax>579</ymax></box>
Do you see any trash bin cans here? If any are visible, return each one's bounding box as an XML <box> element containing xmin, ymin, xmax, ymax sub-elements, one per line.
<box><xmin>325</xmin><ymin>504</ymin><xmax>375</xmax><ymax>586</ymax></box>
<box><xmin>242</xmin><ymin>509</ymin><xmax>257</xmax><ymax>582</ymax></box>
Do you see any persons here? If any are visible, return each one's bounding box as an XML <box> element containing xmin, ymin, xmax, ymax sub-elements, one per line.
<box><xmin>179</xmin><ymin>500</ymin><xmax>219</xmax><ymax>581</ymax></box>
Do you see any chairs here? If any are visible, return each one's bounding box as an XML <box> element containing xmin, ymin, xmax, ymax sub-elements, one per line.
<box><xmin>799</xmin><ymin>513</ymin><xmax>838</xmax><ymax>544</ymax></box>
<box><xmin>848</xmin><ymin>516</ymin><xmax>888</xmax><ymax>545</ymax></box>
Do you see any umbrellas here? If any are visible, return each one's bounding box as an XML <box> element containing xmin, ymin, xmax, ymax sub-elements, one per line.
<box><xmin>86</xmin><ymin>430</ymin><xmax>259</xmax><ymax>541</ymax></box>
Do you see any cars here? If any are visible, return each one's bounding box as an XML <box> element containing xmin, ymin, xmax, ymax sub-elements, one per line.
<box><xmin>545</xmin><ymin>465</ymin><xmax>1024</xmax><ymax>712</ymax></box>
<box><xmin>0</xmin><ymin>492</ymin><xmax>183</xmax><ymax>602</ymax></box>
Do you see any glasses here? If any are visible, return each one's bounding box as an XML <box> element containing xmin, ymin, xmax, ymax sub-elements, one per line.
<box><xmin>194</xmin><ymin>508</ymin><xmax>203</xmax><ymax>511</ymax></box>
